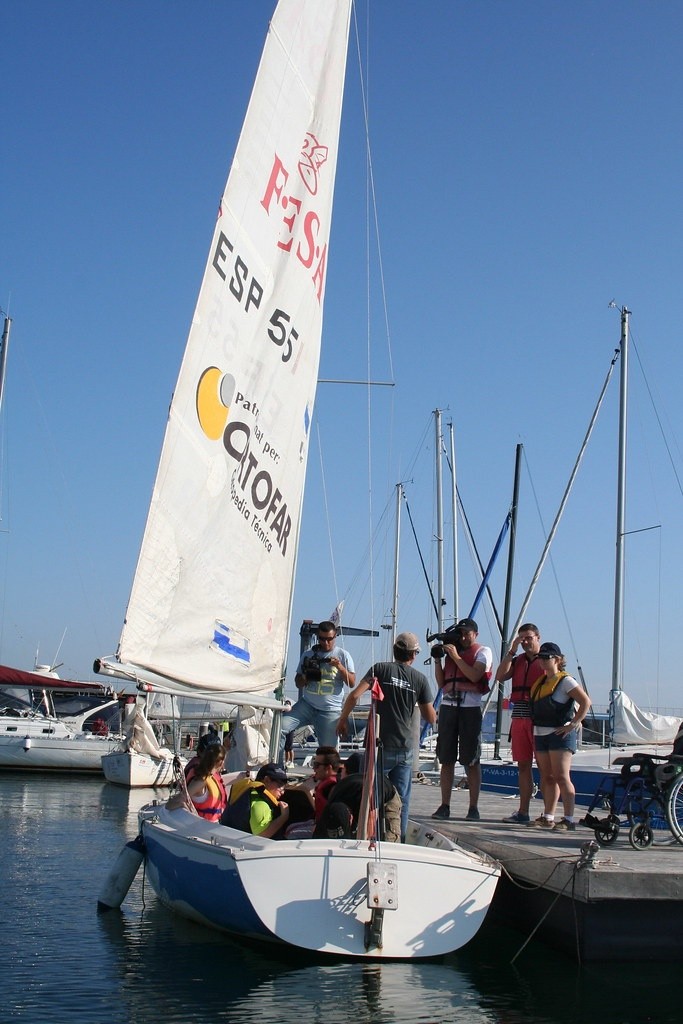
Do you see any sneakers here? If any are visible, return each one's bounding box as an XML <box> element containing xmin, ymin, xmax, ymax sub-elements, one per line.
<box><xmin>527</xmin><ymin>813</ymin><xmax>555</xmax><ymax>828</ymax></box>
<box><xmin>552</xmin><ymin>817</ymin><xmax>576</xmax><ymax>831</ymax></box>
<box><xmin>432</xmin><ymin>805</ymin><xmax>450</xmax><ymax>819</ymax></box>
<box><xmin>503</xmin><ymin>811</ymin><xmax>530</xmax><ymax>823</ymax></box>
<box><xmin>465</xmin><ymin>809</ymin><xmax>480</xmax><ymax>821</ymax></box>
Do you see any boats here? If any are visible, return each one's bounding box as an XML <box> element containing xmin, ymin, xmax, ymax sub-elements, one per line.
<box><xmin>0</xmin><ymin>625</ymin><xmax>162</xmax><ymax>775</ymax></box>
<box><xmin>100</xmin><ymin>689</ymin><xmax>189</xmax><ymax>789</ymax></box>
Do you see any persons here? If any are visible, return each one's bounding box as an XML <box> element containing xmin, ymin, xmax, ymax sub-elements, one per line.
<box><xmin>164</xmin><ymin>732</ymin><xmax>402</xmax><ymax>843</ymax></box>
<box><xmin>526</xmin><ymin>642</ymin><xmax>591</xmax><ymax>832</ymax></box>
<box><xmin>335</xmin><ymin>631</ymin><xmax>437</xmax><ymax>844</ymax></box>
<box><xmin>278</xmin><ymin>621</ymin><xmax>356</xmax><ymax>772</ymax></box>
<box><xmin>208</xmin><ymin>722</ymin><xmax>229</xmax><ymax>735</ymax></box>
<box><xmin>431</xmin><ymin>618</ymin><xmax>493</xmax><ymax>821</ymax></box>
<box><xmin>497</xmin><ymin>623</ymin><xmax>545</xmax><ymax>828</ymax></box>
<box><xmin>283</xmin><ymin>700</ymin><xmax>295</xmax><ymax>768</ymax></box>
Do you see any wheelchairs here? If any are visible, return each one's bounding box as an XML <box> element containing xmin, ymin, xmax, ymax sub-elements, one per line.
<box><xmin>579</xmin><ymin>723</ymin><xmax>683</xmax><ymax>851</ymax></box>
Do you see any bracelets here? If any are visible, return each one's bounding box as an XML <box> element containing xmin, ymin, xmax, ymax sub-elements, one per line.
<box><xmin>435</xmin><ymin>659</ymin><xmax>441</xmax><ymax>663</ymax></box>
<box><xmin>509</xmin><ymin>649</ymin><xmax>516</xmax><ymax>656</ymax></box>
<box><xmin>571</xmin><ymin>719</ymin><xmax>579</xmax><ymax>728</ymax></box>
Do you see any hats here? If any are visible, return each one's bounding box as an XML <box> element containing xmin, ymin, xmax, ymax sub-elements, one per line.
<box><xmin>395</xmin><ymin>632</ymin><xmax>421</xmax><ymax>652</ymax></box>
<box><xmin>533</xmin><ymin>642</ymin><xmax>561</xmax><ymax>656</ymax></box>
<box><xmin>456</xmin><ymin>618</ymin><xmax>478</xmax><ymax>631</ymax></box>
<box><xmin>197</xmin><ymin>734</ymin><xmax>221</xmax><ymax>752</ymax></box>
<box><xmin>258</xmin><ymin>763</ymin><xmax>289</xmax><ymax>784</ymax></box>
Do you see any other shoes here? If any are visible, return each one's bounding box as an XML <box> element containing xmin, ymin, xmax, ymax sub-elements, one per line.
<box><xmin>286</xmin><ymin>761</ymin><xmax>294</xmax><ymax>768</ymax></box>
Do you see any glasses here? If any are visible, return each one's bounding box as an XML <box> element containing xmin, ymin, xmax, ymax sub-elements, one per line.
<box><xmin>527</xmin><ymin>635</ymin><xmax>536</xmax><ymax>641</ymax></box>
<box><xmin>538</xmin><ymin>654</ymin><xmax>555</xmax><ymax>659</ymax></box>
<box><xmin>415</xmin><ymin>651</ymin><xmax>419</xmax><ymax>654</ymax></box>
<box><xmin>313</xmin><ymin>762</ymin><xmax>326</xmax><ymax>768</ymax></box>
<box><xmin>316</xmin><ymin>636</ymin><xmax>335</xmax><ymax>641</ymax></box>
<box><xmin>275</xmin><ymin>781</ymin><xmax>284</xmax><ymax>787</ymax></box>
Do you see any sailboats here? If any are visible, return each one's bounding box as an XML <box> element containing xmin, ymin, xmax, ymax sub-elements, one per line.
<box><xmin>220</xmin><ymin>297</ymin><xmax>682</xmax><ymax>830</ymax></box>
<box><xmin>93</xmin><ymin>0</ymin><xmax>503</xmax><ymax>967</ymax></box>
